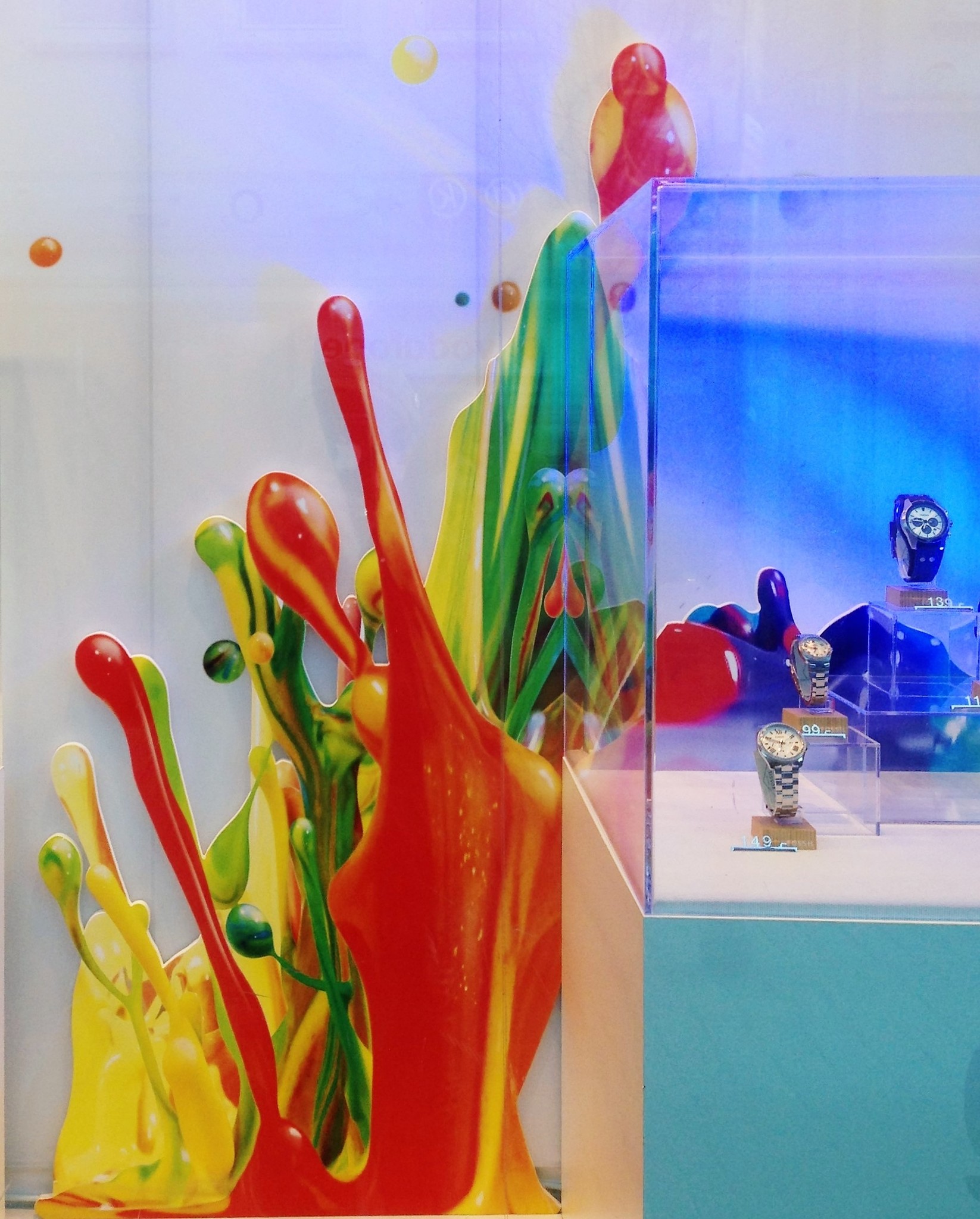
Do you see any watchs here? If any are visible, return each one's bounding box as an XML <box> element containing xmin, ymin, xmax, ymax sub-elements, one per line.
<box><xmin>755</xmin><ymin>722</ymin><xmax>807</xmax><ymax>818</ymax></box>
<box><xmin>888</xmin><ymin>493</ymin><xmax>952</xmax><ymax>582</ymax></box>
<box><xmin>791</xmin><ymin>634</ymin><xmax>833</xmax><ymax>707</ymax></box>
